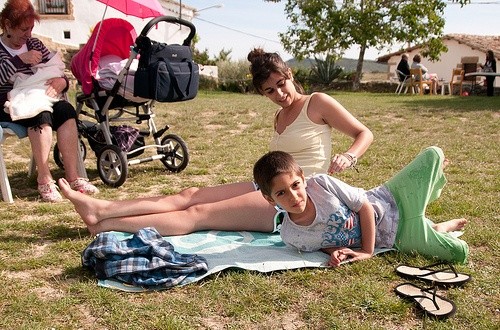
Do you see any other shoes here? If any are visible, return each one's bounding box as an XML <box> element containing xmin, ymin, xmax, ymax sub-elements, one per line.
<box><xmin>37</xmin><ymin>181</ymin><xmax>63</xmax><ymax>203</ymax></box>
<box><xmin>67</xmin><ymin>178</ymin><xmax>98</xmax><ymax>197</ymax></box>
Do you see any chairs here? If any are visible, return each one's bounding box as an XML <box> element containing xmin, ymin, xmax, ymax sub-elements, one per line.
<box><xmin>444</xmin><ymin>81</ymin><xmax>450</xmax><ymax>94</ymax></box>
<box><xmin>394</xmin><ymin>70</ymin><xmax>414</xmax><ymax>94</ymax></box>
<box><xmin>450</xmin><ymin>63</ymin><xmax>477</xmax><ymax>96</ymax></box>
<box><xmin>0</xmin><ymin>124</ymin><xmax>88</xmax><ymax>202</ymax></box>
<box><xmin>410</xmin><ymin>69</ymin><xmax>432</xmax><ymax>96</ymax></box>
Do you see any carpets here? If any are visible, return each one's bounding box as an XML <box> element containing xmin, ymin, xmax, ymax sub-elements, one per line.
<box><xmin>97</xmin><ymin>229</ymin><xmax>396</xmax><ymax>292</ymax></box>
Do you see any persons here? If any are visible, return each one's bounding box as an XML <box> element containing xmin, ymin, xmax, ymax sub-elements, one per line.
<box><xmin>0</xmin><ymin>0</ymin><xmax>100</xmax><ymax>202</ymax></box>
<box><xmin>4</xmin><ymin>48</ymin><xmax>66</xmax><ymax>122</ymax></box>
<box><xmin>397</xmin><ymin>53</ymin><xmax>420</xmax><ymax>95</ymax></box>
<box><xmin>57</xmin><ymin>44</ymin><xmax>374</xmax><ymax>236</ymax></box>
<box><xmin>479</xmin><ymin>49</ymin><xmax>497</xmax><ymax>95</ymax></box>
<box><xmin>411</xmin><ymin>54</ymin><xmax>442</xmax><ymax>95</ymax></box>
<box><xmin>253</xmin><ymin>146</ymin><xmax>469</xmax><ymax>267</ymax></box>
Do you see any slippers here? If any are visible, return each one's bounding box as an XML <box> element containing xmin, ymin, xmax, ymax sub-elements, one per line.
<box><xmin>394</xmin><ymin>259</ymin><xmax>472</xmax><ymax>286</ymax></box>
<box><xmin>395</xmin><ymin>279</ymin><xmax>458</xmax><ymax>319</ymax></box>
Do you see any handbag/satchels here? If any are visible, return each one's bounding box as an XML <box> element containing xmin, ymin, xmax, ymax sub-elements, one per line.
<box><xmin>134</xmin><ymin>45</ymin><xmax>199</xmax><ymax>102</ymax></box>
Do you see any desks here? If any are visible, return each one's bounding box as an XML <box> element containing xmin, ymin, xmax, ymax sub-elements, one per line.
<box><xmin>431</xmin><ymin>78</ymin><xmax>444</xmax><ymax>95</ymax></box>
<box><xmin>465</xmin><ymin>72</ymin><xmax>500</xmax><ymax>96</ymax></box>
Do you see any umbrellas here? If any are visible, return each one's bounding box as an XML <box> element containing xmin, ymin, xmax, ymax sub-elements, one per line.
<box><xmin>88</xmin><ymin>0</ymin><xmax>166</xmax><ymax>65</ymax></box>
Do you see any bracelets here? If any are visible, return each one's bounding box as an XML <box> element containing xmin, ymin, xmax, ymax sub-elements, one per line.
<box><xmin>344</xmin><ymin>153</ymin><xmax>357</xmax><ymax>169</ymax></box>
